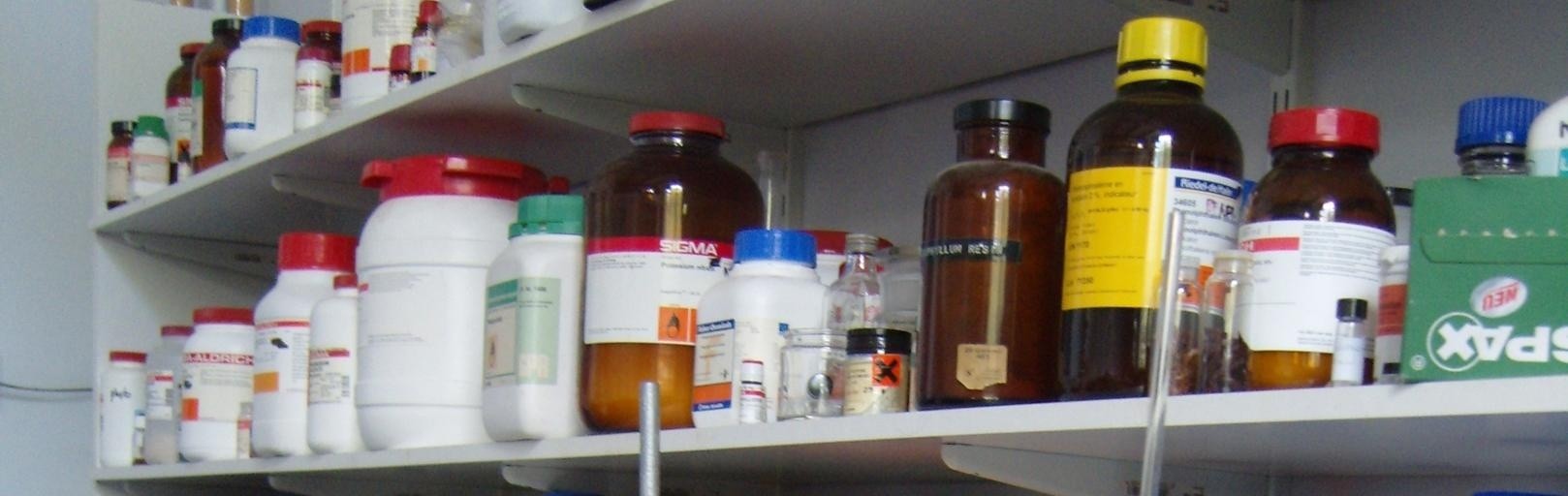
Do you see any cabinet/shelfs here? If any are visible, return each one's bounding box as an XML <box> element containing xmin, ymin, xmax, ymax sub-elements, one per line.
<box><xmin>94</xmin><ymin>1</ymin><xmax>1566</xmax><ymax>485</ymax></box>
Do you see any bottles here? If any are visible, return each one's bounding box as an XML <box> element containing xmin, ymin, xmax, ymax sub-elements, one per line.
<box><xmin>96</xmin><ymin>0</ymin><xmax>1551</xmax><ymax>468</ymax></box>
